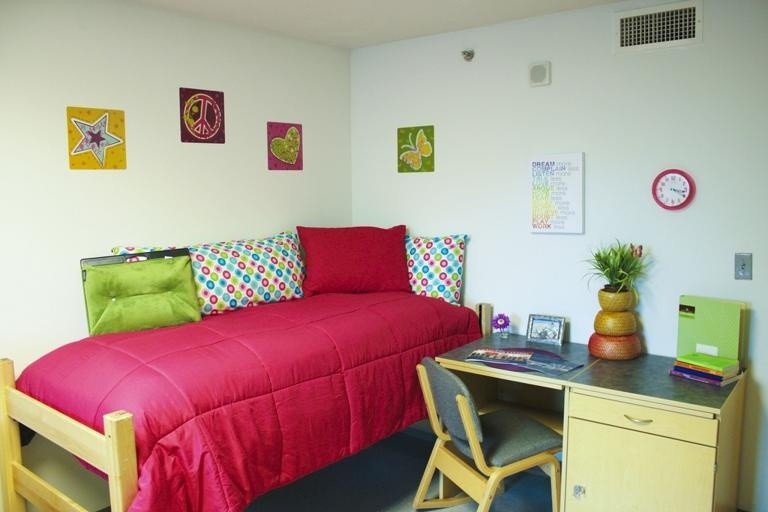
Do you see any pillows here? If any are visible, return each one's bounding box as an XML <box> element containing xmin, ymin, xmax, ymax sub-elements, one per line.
<box><xmin>296</xmin><ymin>224</ymin><xmax>411</xmax><ymax>295</ymax></box>
<box><xmin>82</xmin><ymin>253</ymin><xmax>202</xmax><ymax>337</ymax></box>
<box><xmin>403</xmin><ymin>232</ymin><xmax>467</xmax><ymax>305</ymax></box>
<box><xmin>188</xmin><ymin>232</ymin><xmax>304</xmax><ymax>316</ymax></box>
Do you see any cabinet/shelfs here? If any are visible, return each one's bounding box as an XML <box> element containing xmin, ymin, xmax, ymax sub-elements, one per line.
<box><xmin>558</xmin><ymin>387</ymin><xmax>719</xmax><ymax>511</ymax></box>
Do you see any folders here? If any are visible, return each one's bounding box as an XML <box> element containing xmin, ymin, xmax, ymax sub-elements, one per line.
<box><xmin>677</xmin><ymin>295</ymin><xmax>751</xmax><ymax>366</ymax></box>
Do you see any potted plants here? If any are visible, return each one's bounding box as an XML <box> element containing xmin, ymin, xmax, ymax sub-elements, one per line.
<box><xmin>588</xmin><ymin>244</ymin><xmax>650</xmax><ymax>361</ymax></box>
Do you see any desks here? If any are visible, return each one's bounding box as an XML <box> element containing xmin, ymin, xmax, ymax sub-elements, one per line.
<box><xmin>434</xmin><ymin>332</ymin><xmax>752</xmax><ymax>511</ymax></box>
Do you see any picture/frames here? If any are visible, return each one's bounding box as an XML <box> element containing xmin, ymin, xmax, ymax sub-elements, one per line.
<box><xmin>525</xmin><ymin>314</ymin><xmax>566</xmax><ymax>346</ymax></box>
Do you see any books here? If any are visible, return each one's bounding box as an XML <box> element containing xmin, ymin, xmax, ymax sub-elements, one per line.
<box><xmin>669</xmin><ymin>352</ymin><xmax>744</xmax><ymax>387</ymax></box>
<box><xmin>463</xmin><ymin>343</ymin><xmax>534</xmax><ymax>367</ymax></box>
<box><xmin>513</xmin><ymin>353</ymin><xmax>584</xmax><ymax>376</ymax></box>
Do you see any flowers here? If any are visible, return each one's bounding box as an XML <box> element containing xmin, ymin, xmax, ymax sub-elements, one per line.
<box><xmin>490</xmin><ymin>313</ymin><xmax>510</xmax><ymax>332</ymax></box>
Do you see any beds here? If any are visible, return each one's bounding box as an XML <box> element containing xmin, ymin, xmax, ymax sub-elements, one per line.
<box><xmin>0</xmin><ymin>292</ymin><xmax>495</xmax><ymax>512</ymax></box>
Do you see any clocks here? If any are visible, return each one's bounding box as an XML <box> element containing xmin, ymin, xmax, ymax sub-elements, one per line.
<box><xmin>652</xmin><ymin>169</ymin><xmax>696</xmax><ymax>211</ymax></box>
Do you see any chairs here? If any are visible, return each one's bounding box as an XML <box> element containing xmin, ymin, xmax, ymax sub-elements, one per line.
<box><xmin>412</xmin><ymin>356</ymin><xmax>563</xmax><ymax>511</ymax></box>
